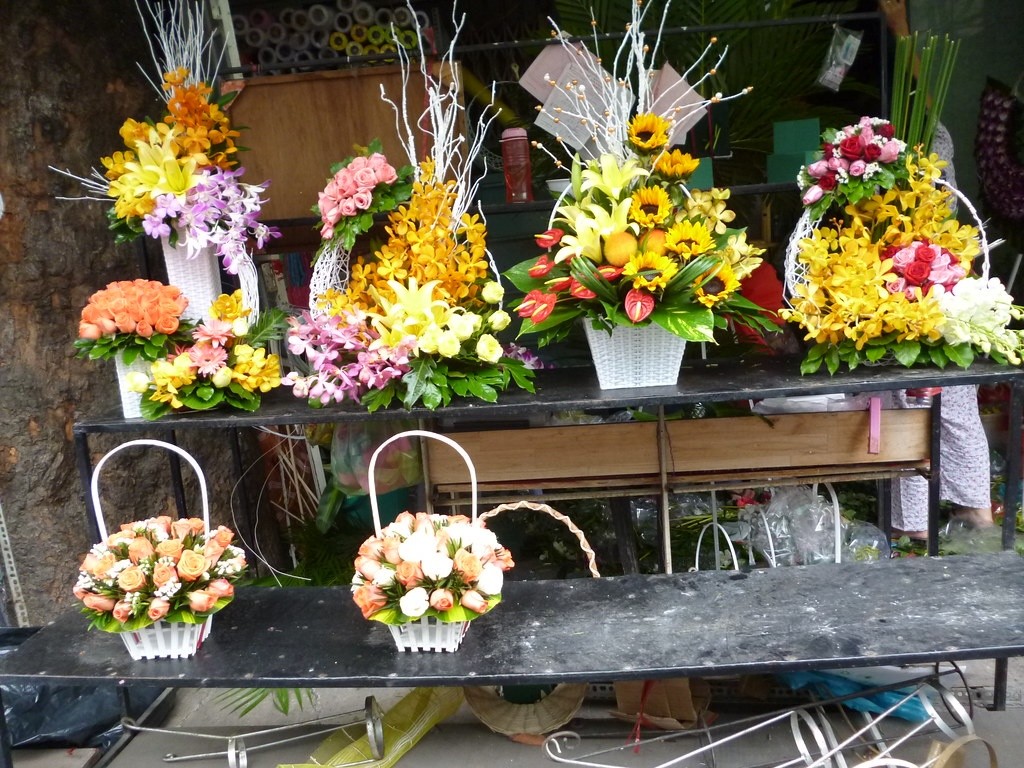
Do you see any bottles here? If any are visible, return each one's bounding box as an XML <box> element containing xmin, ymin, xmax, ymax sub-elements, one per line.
<box><xmin>501</xmin><ymin>127</ymin><xmax>530</xmax><ymax>202</ymax></box>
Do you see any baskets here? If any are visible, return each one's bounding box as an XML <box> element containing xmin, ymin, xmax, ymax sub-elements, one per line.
<box><xmin>307</xmin><ymin>193</ymin><xmax>503</xmax><ymax>394</ymax></box>
<box><xmin>462</xmin><ymin>681</ymin><xmax>586</xmax><ymax>740</ymax></box>
<box><xmin>113</xmin><ymin>339</ymin><xmax>222</xmax><ymax>418</ymax></box>
<box><xmin>368</xmin><ymin>429</ymin><xmax>477</xmax><ymax>651</ymax></box>
<box><xmin>547</xmin><ymin>164</ymin><xmax>706</xmax><ymax>391</ymax></box>
<box><xmin>781</xmin><ymin>168</ymin><xmax>991</xmax><ymax>367</ymax></box>
<box><xmin>85</xmin><ymin>440</ymin><xmax>212</xmax><ymax>662</ymax></box>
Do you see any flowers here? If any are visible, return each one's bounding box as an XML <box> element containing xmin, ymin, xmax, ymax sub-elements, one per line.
<box><xmin>502</xmin><ymin>0</ymin><xmax>783</xmax><ymax>391</ymax></box>
<box><xmin>282</xmin><ymin>0</ymin><xmax>535</xmax><ymax>413</ymax></box>
<box><xmin>72</xmin><ymin>516</ymin><xmax>248</xmax><ymax>633</ymax></box>
<box><xmin>73</xmin><ymin>277</ymin><xmax>194</xmax><ymax>366</ymax></box>
<box><xmin>48</xmin><ymin>0</ymin><xmax>282</xmax><ymax>274</ymax></box>
<box><xmin>125</xmin><ymin>292</ymin><xmax>288</xmax><ymax>420</ymax></box>
<box><xmin>777</xmin><ymin>33</ymin><xmax>1024</xmax><ymax>377</ymax></box>
<box><xmin>350</xmin><ymin>511</ymin><xmax>516</xmax><ymax>626</ymax></box>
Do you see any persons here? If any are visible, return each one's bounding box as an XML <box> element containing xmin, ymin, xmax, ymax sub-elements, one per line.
<box><xmin>801</xmin><ymin>0</ymin><xmax>991</xmax><ymax>540</ymax></box>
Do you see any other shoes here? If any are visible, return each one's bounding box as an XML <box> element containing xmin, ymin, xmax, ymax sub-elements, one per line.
<box><xmin>891</xmin><ymin>532</ymin><xmax>929</xmax><ymax>553</ymax></box>
